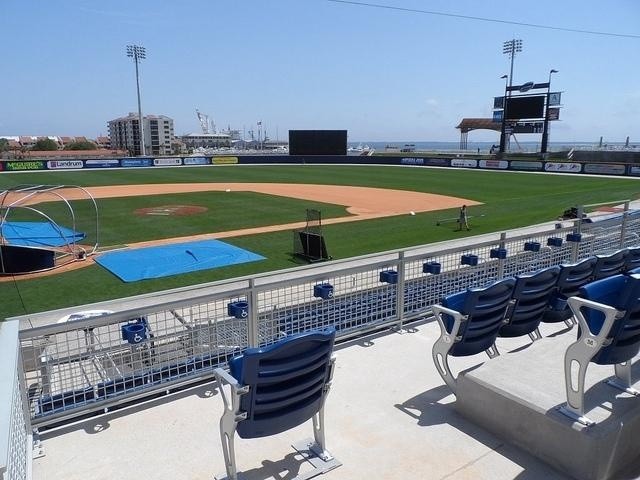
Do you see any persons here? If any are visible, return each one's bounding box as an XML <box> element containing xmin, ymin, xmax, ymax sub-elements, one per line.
<box><xmin>457</xmin><ymin>204</ymin><xmax>472</xmax><ymax>231</ymax></box>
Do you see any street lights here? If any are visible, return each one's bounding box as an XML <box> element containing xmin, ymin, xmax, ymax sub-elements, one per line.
<box><xmin>540</xmin><ymin>69</ymin><xmax>560</xmax><ymax>157</ymax></box>
<box><xmin>499</xmin><ymin>75</ymin><xmax>509</xmax><ymax>152</ymax></box>
<box><xmin>499</xmin><ymin>38</ymin><xmax>522</xmax><ymax>154</ymax></box>
<box><xmin>126</xmin><ymin>42</ymin><xmax>147</xmax><ymax>157</ymax></box>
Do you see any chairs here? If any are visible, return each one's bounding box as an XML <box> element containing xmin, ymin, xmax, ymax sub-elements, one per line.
<box><xmin>212</xmin><ymin>327</ymin><xmax>343</xmax><ymax>480</ymax></box>
<box><xmin>431</xmin><ymin>245</ymin><xmax>639</xmax><ymax>428</ymax></box>
